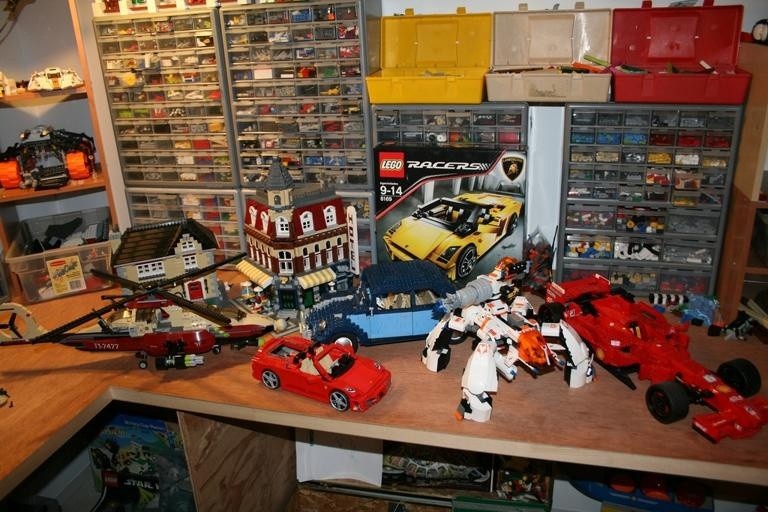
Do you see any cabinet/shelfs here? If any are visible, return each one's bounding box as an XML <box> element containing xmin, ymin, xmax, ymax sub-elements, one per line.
<box><xmin>0</xmin><ymin>0</ymin><xmax>768</xmax><ymax>313</ymax></box>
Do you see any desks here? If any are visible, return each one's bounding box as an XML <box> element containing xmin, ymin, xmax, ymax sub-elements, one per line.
<box><xmin>1</xmin><ymin>254</ymin><xmax>768</xmax><ymax>510</ymax></box>
